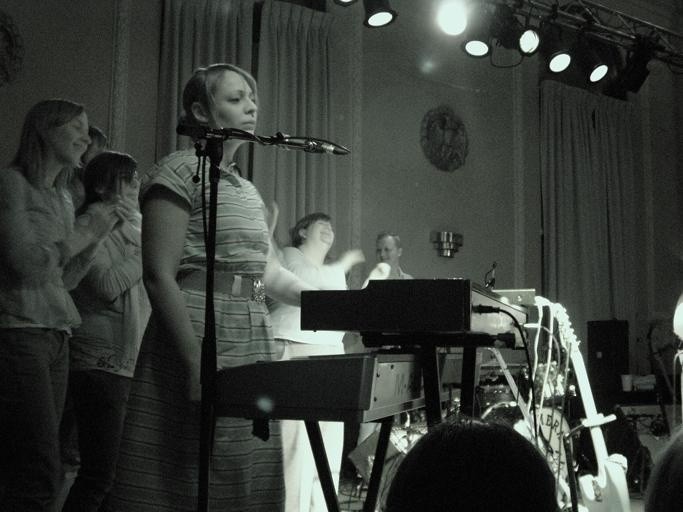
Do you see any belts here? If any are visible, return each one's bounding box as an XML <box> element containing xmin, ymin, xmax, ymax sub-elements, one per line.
<box><xmin>178</xmin><ymin>269</ymin><xmax>266</xmax><ymax>303</ymax></box>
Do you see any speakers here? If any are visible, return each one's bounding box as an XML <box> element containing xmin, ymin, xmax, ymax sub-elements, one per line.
<box><xmin>588</xmin><ymin>320</ymin><xmax>630</xmax><ymax>375</ymax></box>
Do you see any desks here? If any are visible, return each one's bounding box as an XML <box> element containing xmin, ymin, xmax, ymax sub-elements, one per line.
<box><xmin>616</xmin><ymin>391</ymin><xmax>673</xmax><ymax>438</ymax></box>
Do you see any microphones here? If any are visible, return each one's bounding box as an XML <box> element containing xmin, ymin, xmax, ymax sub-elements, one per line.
<box><xmin>275</xmin><ymin>132</ymin><xmax>346</xmax><ymax>158</ymax></box>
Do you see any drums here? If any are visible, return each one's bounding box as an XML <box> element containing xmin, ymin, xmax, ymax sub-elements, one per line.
<box><xmin>529</xmin><ymin>359</ymin><xmax>569</xmax><ymax>404</ymax></box>
<box><xmin>479</xmin><ymin>401</ymin><xmax>573</xmax><ymax>495</ymax></box>
<box><xmin>473</xmin><ymin>381</ymin><xmax>526</xmax><ymax>409</ymax></box>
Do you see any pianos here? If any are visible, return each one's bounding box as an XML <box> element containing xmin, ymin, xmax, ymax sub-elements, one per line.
<box><xmin>216</xmin><ymin>344</ymin><xmax>530</xmax><ymax>422</ymax></box>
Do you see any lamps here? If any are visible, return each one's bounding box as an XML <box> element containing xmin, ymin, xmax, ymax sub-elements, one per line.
<box><xmin>331</xmin><ymin>0</ymin><xmax>613</xmax><ymax>83</ymax></box>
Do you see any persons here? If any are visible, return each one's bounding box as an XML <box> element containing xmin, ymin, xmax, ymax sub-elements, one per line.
<box><xmin>67</xmin><ymin>124</ymin><xmax>107</xmax><ymax>219</ymax></box>
<box><xmin>0</xmin><ymin>99</ymin><xmax>93</xmax><ymax>511</ymax></box>
<box><xmin>266</xmin><ymin>211</ymin><xmax>390</xmax><ymax>512</ymax></box>
<box><xmin>374</xmin><ymin>231</ymin><xmax>416</xmax><ymax>280</ymax></box>
<box><xmin>101</xmin><ymin>64</ymin><xmax>285</xmax><ymax>512</ymax></box>
<box><xmin>374</xmin><ymin>414</ymin><xmax>555</xmax><ymax>511</ymax></box>
<box><xmin>62</xmin><ymin>150</ymin><xmax>153</xmax><ymax>511</ymax></box>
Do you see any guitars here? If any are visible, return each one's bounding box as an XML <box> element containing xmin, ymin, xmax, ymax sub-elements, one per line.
<box><xmin>552</xmin><ymin>302</ymin><xmax>630</xmax><ymax>511</ymax></box>
